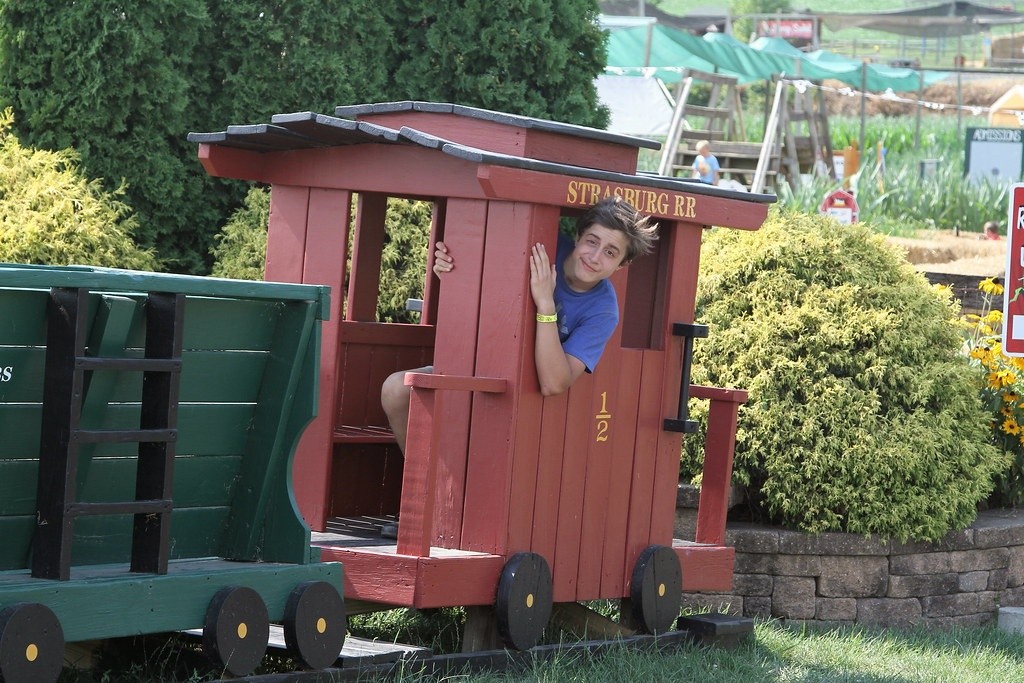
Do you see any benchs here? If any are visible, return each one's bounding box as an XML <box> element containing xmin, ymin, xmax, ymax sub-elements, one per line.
<box><xmin>333</xmin><ymin>425</ymin><xmax>396</xmax><ymax>446</ymax></box>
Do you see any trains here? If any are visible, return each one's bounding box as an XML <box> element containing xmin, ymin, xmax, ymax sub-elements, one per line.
<box><xmin>0</xmin><ymin>99</ymin><xmax>758</xmax><ymax>683</ymax></box>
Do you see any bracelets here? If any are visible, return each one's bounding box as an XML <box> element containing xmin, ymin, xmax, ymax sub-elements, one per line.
<box><xmin>535</xmin><ymin>314</ymin><xmax>559</xmax><ymax>324</ymax></box>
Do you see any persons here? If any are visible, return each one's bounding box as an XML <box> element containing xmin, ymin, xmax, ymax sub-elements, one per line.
<box><xmin>381</xmin><ymin>198</ymin><xmax>647</xmax><ymax>538</ymax></box>
<box><xmin>983</xmin><ymin>223</ymin><xmax>1000</xmax><ymax>240</ymax></box>
<box><xmin>692</xmin><ymin>140</ymin><xmax>718</xmax><ymax>185</ymax></box>
